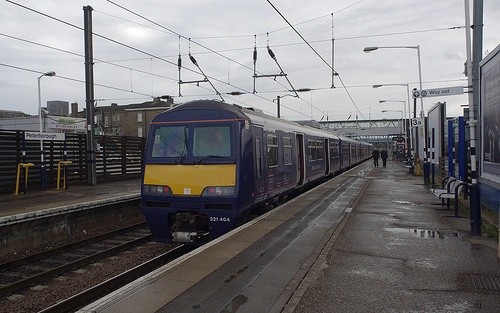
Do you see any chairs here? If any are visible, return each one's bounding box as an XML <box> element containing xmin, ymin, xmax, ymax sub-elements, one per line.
<box><xmin>400</xmin><ymin>154</ymin><xmax>417</xmax><ymax>176</ymax></box>
<box><xmin>428</xmin><ymin>176</ymin><xmax>468</xmax><ymax>218</ymax></box>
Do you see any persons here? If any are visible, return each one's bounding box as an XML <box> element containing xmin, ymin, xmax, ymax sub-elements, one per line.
<box><xmin>372</xmin><ymin>150</ymin><xmax>380</xmax><ymax>167</ymax></box>
<box><xmin>409</xmin><ymin>153</ymin><xmax>422</xmax><ymax>176</ymax></box>
<box><xmin>381</xmin><ymin>148</ymin><xmax>388</xmax><ymax>167</ymax></box>
<box><xmin>391</xmin><ymin>149</ymin><xmax>400</xmax><ymax>161</ymax></box>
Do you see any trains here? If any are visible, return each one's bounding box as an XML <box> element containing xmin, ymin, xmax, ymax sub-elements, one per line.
<box><xmin>141</xmin><ymin>34</ymin><xmax>378</xmax><ymax>244</ymax></box>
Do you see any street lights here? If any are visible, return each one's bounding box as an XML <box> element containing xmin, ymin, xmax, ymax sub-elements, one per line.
<box><xmin>378</xmin><ymin>99</ymin><xmax>408</xmax><ymax>150</ymax></box>
<box><xmin>37</xmin><ymin>71</ymin><xmax>56</xmax><ymax>190</ymax></box>
<box><xmin>382</xmin><ymin>109</ymin><xmax>405</xmax><ymax>133</ymax></box>
<box><xmin>363</xmin><ymin>45</ymin><xmax>427</xmax><ymax>162</ymax></box>
<box><xmin>373</xmin><ymin>83</ymin><xmax>413</xmax><ymax>150</ymax></box>
